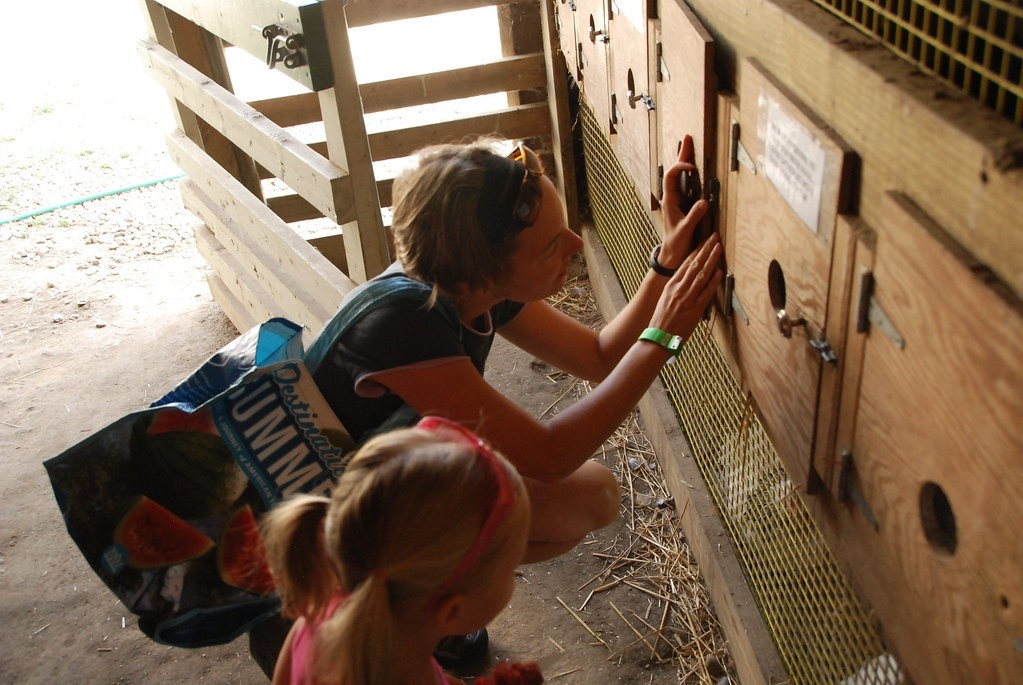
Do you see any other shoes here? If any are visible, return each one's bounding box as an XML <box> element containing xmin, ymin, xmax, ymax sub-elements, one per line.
<box><xmin>435</xmin><ymin>621</ymin><xmax>488</xmax><ymax>662</ymax></box>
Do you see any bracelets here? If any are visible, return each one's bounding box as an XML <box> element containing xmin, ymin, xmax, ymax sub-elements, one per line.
<box><xmin>649</xmin><ymin>244</ymin><xmax>678</xmax><ymax>277</ymax></box>
<box><xmin>638</xmin><ymin>327</ymin><xmax>684</xmax><ymax>364</ymax></box>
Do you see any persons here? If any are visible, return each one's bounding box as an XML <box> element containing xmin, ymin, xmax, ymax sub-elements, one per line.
<box><xmin>248</xmin><ymin>134</ymin><xmax>723</xmax><ymax>682</ymax></box>
<box><xmin>269</xmin><ymin>426</ymin><xmax>545</xmax><ymax>685</ymax></box>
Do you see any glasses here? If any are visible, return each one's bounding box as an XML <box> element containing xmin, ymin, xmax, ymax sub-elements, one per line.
<box><xmin>488</xmin><ymin>141</ymin><xmax>544</xmax><ymax>253</ymax></box>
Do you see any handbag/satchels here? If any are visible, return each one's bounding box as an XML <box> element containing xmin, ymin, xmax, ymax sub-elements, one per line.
<box><xmin>42</xmin><ymin>278</ymin><xmax>464</xmax><ymax>651</ymax></box>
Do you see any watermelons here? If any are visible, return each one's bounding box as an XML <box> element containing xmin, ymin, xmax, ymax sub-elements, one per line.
<box><xmin>108</xmin><ymin>408</ymin><xmax>279</xmax><ymax>596</ymax></box>
